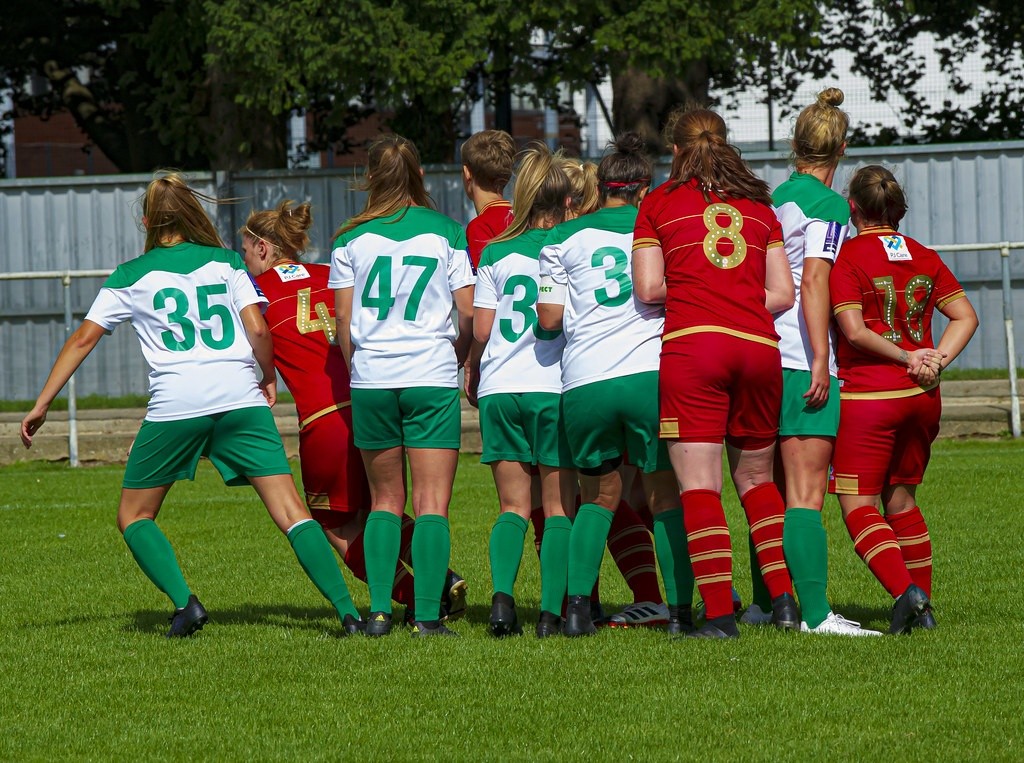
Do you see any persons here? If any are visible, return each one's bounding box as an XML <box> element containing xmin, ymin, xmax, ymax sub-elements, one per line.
<box><xmin>767</xmin><ymin>87</ymin><xmax>883</xmax><ymax>639</ymax></box>
<box><xmin>460</xmin><ymin>127</ymin><xmax>776</xmax><ymax>638</ymax></box>
<box><xmin>829</xmin><ymin>165</ymin><xmax>982</xmax><ymax>640</ymax></box>
<box><xmin>19</xmin><ymin>173</ymin><xmax>369</xmax><ymax>637</ymax></box>
<box><xmin>630</xmin><ymin>110</ymin><xmax>799</xmax><ymax>642</ymax></box>
<box><xmin>241</xmin><ymin>204</ymin><xmax>470</xmax><ymax>629</ymax></box>
<box><xmin>323</xmin><ymin>134</ymin><xmax>475</xmax><ymax>640</ymax></box>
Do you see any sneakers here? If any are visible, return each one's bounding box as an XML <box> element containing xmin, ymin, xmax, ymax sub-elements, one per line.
<box><xmin>695</xmin><ymin>588</ymin><xmax>741</xmax><ymax>622</ymax></box>
<box><xmin>166</xmin><ymin>594</ymin><xmax>209</xmax><ymax>638</ymax></box>
<box><xmin>595</xmin><ymin>601</ymin><xmax>669</xmax><ymax>628</ymax></box>
<box><xmin>739</xmin><ymin>603</ymin><xmax>773</xmax><ymax>625</ymax></box>
<box><xmin>666</xmin><ymin>602</ymin><xmax>698</xmax><ymax>634</ymax></box>
<box><xmin>411</xmin><ymin>621</ymin><xmax>457</xmax><ymax>638</ymax></box>
<box><xmin>367</xmin><ymin>611</ymin><xmax>392</xmax><ymax>636</ymax></box>
<box><xmin>537</xmin><ymin>610</ymin><xmax>561</xmax><ymax>637</ymax></box>
<box><xmin>561</xmin><ymin>596</ymin><xmax>604</xmax><ymax>628</ymax></box>
<box><xmin>687</xmin><ymin>611</ymin><xmax>740</xmax><ymax>638</ymax></box>
<box><xmin>801</xmin><ymin>610</ymin><xmax>884</xmax><ymax>637</ymax></box>
<box><xmin>342</xmin><ymin>613</ymin><xmax>366</xmax><ymax>637</ymax></box>
<box><xmin>889</xmin><ymin>583</ymin><xmax>937</xmax><ymax>636</ymax></box>
<box><xmin>404</xmin><ymin>607</ymin><xmax>448</xmax><ymax>626</ymax></box>
<box><xmin>443</xmin><ymin>572</ymin><xmax>468</xmax><ymax>615</ymax></box>
<box><xmin>564</xmin><ymin>595</ymin><xmax>597</xmax><ymax>638</ymax></box>
<box><xmin>490</xmin><ymin>591</ymin><xmax>524</xmax><ymax>637</ymax></box>
<box><xmin>772</xmin><ymin>592</ymin><xmax>800</xmax><ymax>631</ymax></box>
